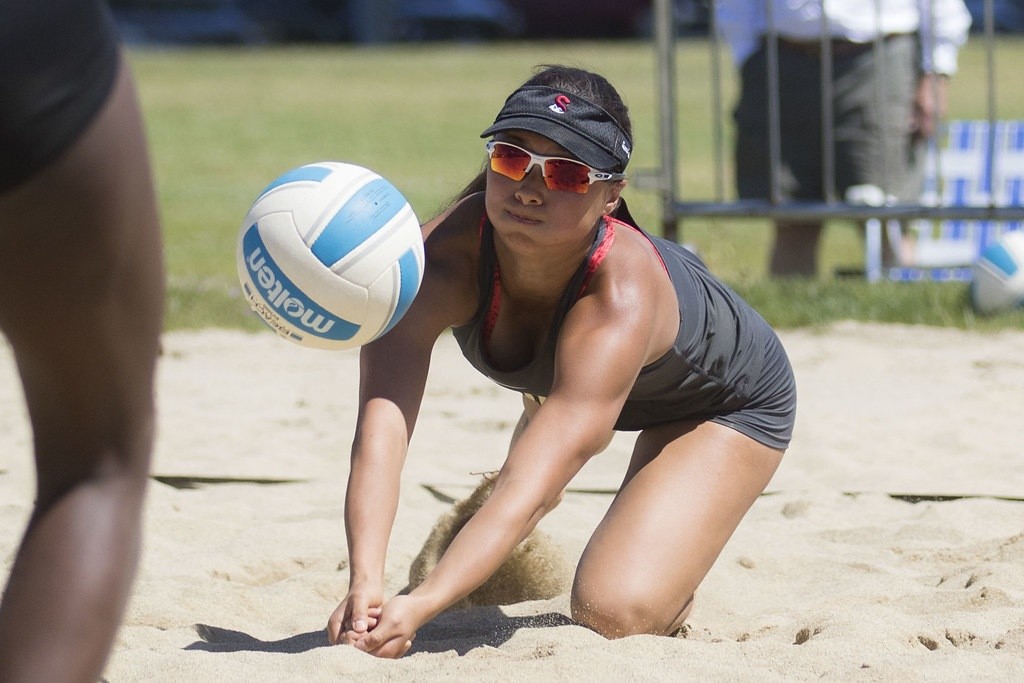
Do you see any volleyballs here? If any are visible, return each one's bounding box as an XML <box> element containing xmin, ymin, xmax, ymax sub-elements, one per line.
<box><xmin>234</xmin><ymin>162</ymin><xmax>426</xmax><ymax>354</ymax></box>
<box><xmin>973</xmin><ymin>228</ymin><xmax>1024</xmax><ymax>313</ymax></box>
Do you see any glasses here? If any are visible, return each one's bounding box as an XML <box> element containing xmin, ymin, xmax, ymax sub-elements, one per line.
<box><xmin>485</xmin><ymin>140</ymin><xmax>631</xmax><ymax>195</ymax></box>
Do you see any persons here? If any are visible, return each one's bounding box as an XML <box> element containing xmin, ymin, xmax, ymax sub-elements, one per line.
<box><xmin>4</xmin><ymin>0</ymin><xmax>165</xmax><ymax>682</ymax></box>
<box><xmin>712</xmin><ymin>0</ymin><xmax>973</xmax><ymax>273</ymax></box>
<box><xmin>326</xmin><ymin>67</ymin><xmax>797</xmax><ymax>658</ymax></box>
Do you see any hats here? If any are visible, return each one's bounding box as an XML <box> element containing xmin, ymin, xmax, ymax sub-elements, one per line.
<box><xmin>480</xmin><ymin>85</ymin><xmax>635</xmax><ymax>175</ymax></box>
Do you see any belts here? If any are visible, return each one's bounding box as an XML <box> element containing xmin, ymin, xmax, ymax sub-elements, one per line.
<box><xmin>760</xmin><ymin>33</ymin><xmax>900</xmax><ymax>58</ymax></box>
